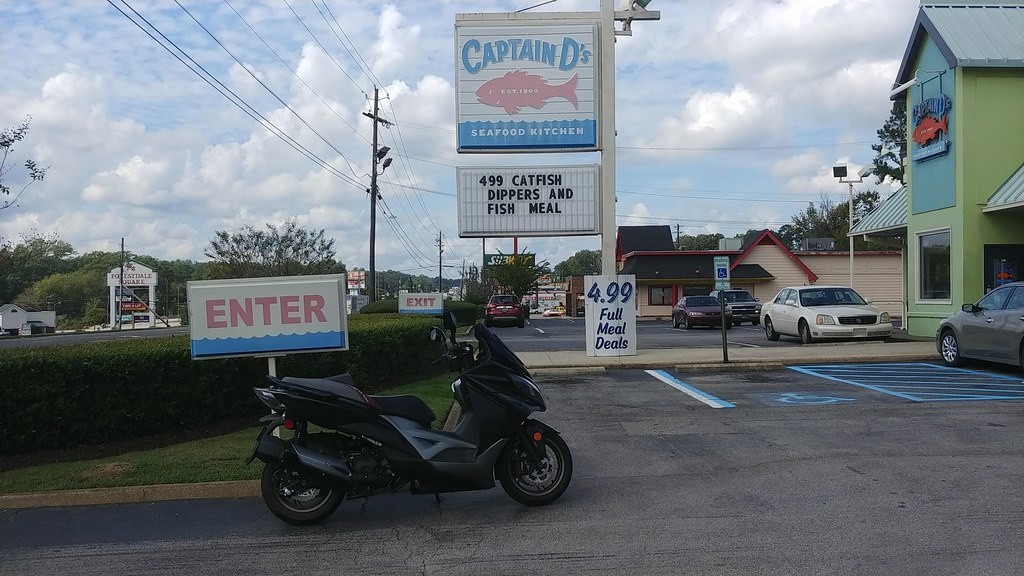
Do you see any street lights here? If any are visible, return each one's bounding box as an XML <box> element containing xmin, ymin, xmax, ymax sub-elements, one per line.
<box><xmin>833</xmin><ymin>164</ymin><xmax>877</xmax><ymax>286</ymax></box>
<box><xmin>369</xmin><ymin>144</ymin><xmax>393</xmax><ymax>304</ymax></box>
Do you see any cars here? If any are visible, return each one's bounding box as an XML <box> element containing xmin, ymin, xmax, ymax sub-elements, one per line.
<box><xmin>671</xmin><ymin>296</ymin><xmax>733</xmax><ymax>329</ymax></box>
<box><xmin>935</xmin><ymin>282</ymin><xmax>1024</xmax><ymax>373</ymax></box>
<box><xmin>529</xmin><ymin>307</ymin><xmax>566</xmax><ymax>316</ymax></box>
<box><xmin>760</xmin><ymin>286</ymin><xmax>893</xmax><ymax>343</ymax></box>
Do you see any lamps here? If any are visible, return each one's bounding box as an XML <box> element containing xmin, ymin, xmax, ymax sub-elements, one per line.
<box><xmin>889</xmin><ymin>68</ymin><xmax>946</xmax><ymax>96</ymax></box>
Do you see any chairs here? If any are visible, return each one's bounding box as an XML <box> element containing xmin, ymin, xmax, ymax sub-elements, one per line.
<box><xmin>801</xmin><ymin>295</ymin><xmax>839</xmax><ymax>305</ymax></box>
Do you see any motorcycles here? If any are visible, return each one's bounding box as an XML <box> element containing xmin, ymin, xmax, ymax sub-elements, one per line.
<box><xmin>245</xmin><ymin>308</ymin><xmax>573</xmax><ymax>526</ymax></box>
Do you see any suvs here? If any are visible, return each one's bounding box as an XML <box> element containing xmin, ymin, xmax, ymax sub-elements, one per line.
<box><xmin>709</xmin><ymin>290</ymin><xmax>763</xmax><ymax>326</ymax></box>
<box><xmin>484</xmin><ymin>293</ymin><xmax>525</xmax><ymax>328</ymax></box>
